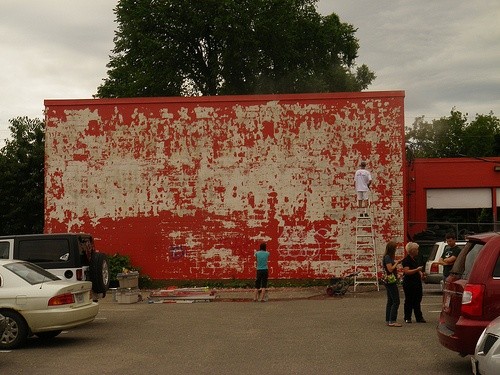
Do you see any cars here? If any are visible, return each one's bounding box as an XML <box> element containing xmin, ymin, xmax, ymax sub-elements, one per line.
<box><xmin>436</xmin><ymin>232</ymin><xmax>500</xmax><ymax>358</ymax></box>
<box><xmin>470</xmin><ymin>316</ymin><xmax>499</xmax><ymax>375</ymax></box>
<box><xmin>0</xmin><ymin>259</ymin><xmax>99</xmax><ymax>350</ymax></box>
<box><xmin>422</xmin><ymin>241</ymin><xmax>467</xmax><ymax>284</ymax></box>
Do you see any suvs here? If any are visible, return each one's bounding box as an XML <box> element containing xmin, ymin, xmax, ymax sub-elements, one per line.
<box><xmin>0</xmin><ymin>233</ymin><xmax>110</xmax><ymax>294</ymax></box>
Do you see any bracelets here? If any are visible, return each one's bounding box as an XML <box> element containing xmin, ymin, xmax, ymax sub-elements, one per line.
<box><xmin>396</xmin><ymin>262</ymin><xmax>398</xmax><ymax>264</ymax></box>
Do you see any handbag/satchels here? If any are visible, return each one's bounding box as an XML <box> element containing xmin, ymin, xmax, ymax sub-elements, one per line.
<box><xmin>381</xmin><ymin>255</ymin><xmax>397</xmax><ymax>283</ymax></box>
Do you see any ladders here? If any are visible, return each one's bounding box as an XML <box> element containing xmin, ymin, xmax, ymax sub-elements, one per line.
<box><xmin>353</xmin><ymin>183</ymin><xmax>379</xmax><ymax>293</ymax></box>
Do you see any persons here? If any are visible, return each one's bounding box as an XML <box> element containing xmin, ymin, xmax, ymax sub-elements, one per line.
<box><xmin>254</xmin><ymin>243</ymin><xmax>270</xmax><ymax>302</ymax></box>
<box><xmin>438</xmin><ymin>232</ymin><xmax>462</xmax><ymax>284</ymax></box>
<box><xmin>464</xmin><ymin>232</ymin><xmax>474</xmax><ymax>241</ymax></box>
<box><xmin>353</xmin><ymin>161</ymin><xmax>372</xmax><ymax>217</ymax></box>
<box><xmin>401</xmin><ymin>242</ymin><xmax>426</xmax><ymax>323</ymax></box>
<box><xmin>382</xmin><ymin>242</ymin><xmax>403</xmax><ymax>327</ymax></box>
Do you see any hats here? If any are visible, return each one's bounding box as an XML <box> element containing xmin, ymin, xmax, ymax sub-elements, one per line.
<box><xmin>359</xmin><ymin>162</ymin><xmax>366</xmax><ymax>167</ymax></box>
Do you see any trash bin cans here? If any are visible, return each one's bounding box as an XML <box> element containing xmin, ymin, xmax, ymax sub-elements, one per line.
<box><xmin>117</xmin><ymin>270</ymin><xmax>139</xmax><ymax>289</ymax></box>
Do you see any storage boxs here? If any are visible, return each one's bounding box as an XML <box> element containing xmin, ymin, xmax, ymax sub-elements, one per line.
<box><xmin>113</xmin><ymin>272</ymin><xmax>139</xmax><ymax>304</ymax></box>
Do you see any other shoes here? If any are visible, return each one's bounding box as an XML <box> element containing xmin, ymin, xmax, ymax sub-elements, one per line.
<box><xmin>405</xmin><ymin>319</ymin><xmax>412</xmax><ymax>323</ymax></box>
<box><xmin>419</xmin><ymin>320</ymin><xmax>427</xmax><ymax>323</ymax></box>
<box><xmin>387</xmin><ymin>322</ymin><xmax>402</xmax><ymax>326</ymax></box>
<box><xmin>365</xmin><ymin>213</ymin><xmax>368</xmax><ymax>217</ymax></box>
<box><xmin>360</xmin><ymin>213</ymin><xmax>363</xmax><ymax>217</ymax></box>
